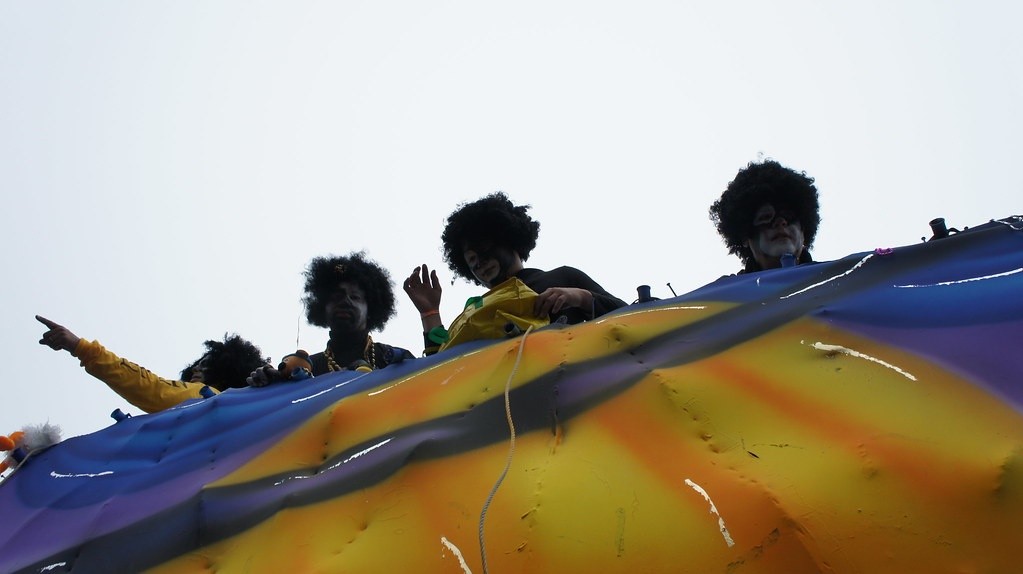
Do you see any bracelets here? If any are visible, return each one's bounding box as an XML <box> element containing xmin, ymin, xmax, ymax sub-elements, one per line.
<box><xmin>420</xmin><ymin>309</ymin><xmax>439</xmax><ymax>318</ymax></box>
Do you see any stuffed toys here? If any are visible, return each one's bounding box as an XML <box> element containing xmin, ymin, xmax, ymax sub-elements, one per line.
<box><xmin>0</xmin><ymin>426</ymin><xmax>62</xmax><ymax>474</ymax></box>
<box><xmin>277</xmin><ymin>349</ymin><xmax>313</xmax><ymax>380</ymax></box>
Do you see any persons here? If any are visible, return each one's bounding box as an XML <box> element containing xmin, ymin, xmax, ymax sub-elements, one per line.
<box><xmin>245</xmin><ymin>251</ymin><xmax>416</xmax><ymax>388</ymax></box>
<box><xmin>34</xmin><ymin>315</ymin><xmax>268</xmax><ymax>413</ymax></box>
<box><xmin>708</xmin><ymin>160</ymin><xmax>821</xmax><ymax>282</ymax></box>
<box><xmin>403</xmin><ymin>191</ymin><xmax>629</xmax><ymax>356</ymax></box>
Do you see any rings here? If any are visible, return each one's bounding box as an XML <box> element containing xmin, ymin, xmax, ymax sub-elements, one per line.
<box><xmin>49</xmin><ymin>337</ymin><xmax>53</xmax><ymax>341</ymax></box>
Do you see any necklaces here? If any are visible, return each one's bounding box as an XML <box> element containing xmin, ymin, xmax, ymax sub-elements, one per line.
<box><xmin>322</xmin><ymin>341</ymin><xmax>376</xmax><ymax>371</ymax></box>
